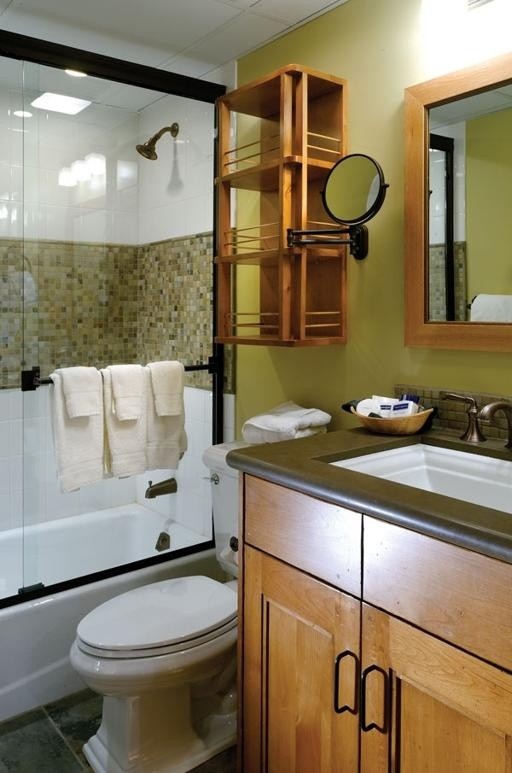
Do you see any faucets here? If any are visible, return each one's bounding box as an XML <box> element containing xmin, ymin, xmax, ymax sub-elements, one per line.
<box><xmin>479</xmin><ymin>397</ymin><xmax>510</xmax><ymax>440</ymax></box>
<box><xmin>143</xmin><ymin>476</ymin><xmax>179</xmax><ymax>497</ymax></box>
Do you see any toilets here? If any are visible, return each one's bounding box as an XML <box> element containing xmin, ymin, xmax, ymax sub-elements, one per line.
<box><xmin>69</xmin><ymin>437</ymin><xmax>246</xmax><ymax>770</ymax></box>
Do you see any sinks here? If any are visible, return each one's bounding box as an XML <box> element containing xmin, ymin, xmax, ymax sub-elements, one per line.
<box><xmin>311</xmin><ymin>436</ymin><xmax>512</xmax><ymax>515</ymax></box>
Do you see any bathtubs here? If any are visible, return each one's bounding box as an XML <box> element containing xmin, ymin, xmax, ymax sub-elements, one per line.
<box><xmin>0</xmin><ymin>503</ymin><xmax>216</xmax><ymax>721</ymax></box>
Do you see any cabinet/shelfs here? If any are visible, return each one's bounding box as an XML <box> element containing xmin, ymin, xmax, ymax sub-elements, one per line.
<box><xmin>211</xmin><ymin>65</ymin><xmax>350</xmax><ymax>347</ymax></box>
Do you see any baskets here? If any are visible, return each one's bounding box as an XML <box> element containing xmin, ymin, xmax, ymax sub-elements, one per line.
<box><xmin>350</xmin><ymin>397</ymin><xmax>434</xmax><ymax>435</ymax></box>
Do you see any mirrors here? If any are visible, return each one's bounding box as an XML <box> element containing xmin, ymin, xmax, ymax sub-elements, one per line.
<box><xmin>321</xmin><ymin>152</ymin><xmax>386</xmax><ymax>225</ymax></box>
<box><xmin>402</xmin><ymin>52</ymin><xmax>512</xmax><ymax>354</ymax></box>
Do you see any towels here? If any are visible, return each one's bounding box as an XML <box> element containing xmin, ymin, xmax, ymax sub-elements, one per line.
<box><xmin>47</xmin><ymin>360</ymin><xmax>189</xmax><ymax>493</ymax></box>
<box><xmin>241</xmin><ymin>399</ymin><xmax>333</xmax><ymax>446</ymax></box>
